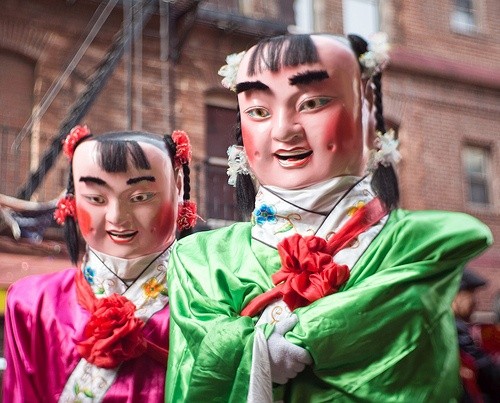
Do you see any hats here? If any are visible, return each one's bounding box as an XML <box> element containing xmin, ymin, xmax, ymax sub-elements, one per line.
<box><xmin>459</xmin><ymin>269</ymin><xmax>486</xmax><ymax>289</ymax></box>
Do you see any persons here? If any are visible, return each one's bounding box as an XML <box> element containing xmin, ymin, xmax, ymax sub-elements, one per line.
<box><xmin>164</xmin><ymin>30</ymin><xmax>494</xmax><ymax>403</ymax></box>
<box><xmin>452</xmin><ymin>270</ymin><xmax>500</xmax><ymax>403</ymax></box>
<box><xmin>0</xmin><ymin>123</ymin><xmax>197</xmax><ymax>403</ymax></box>
